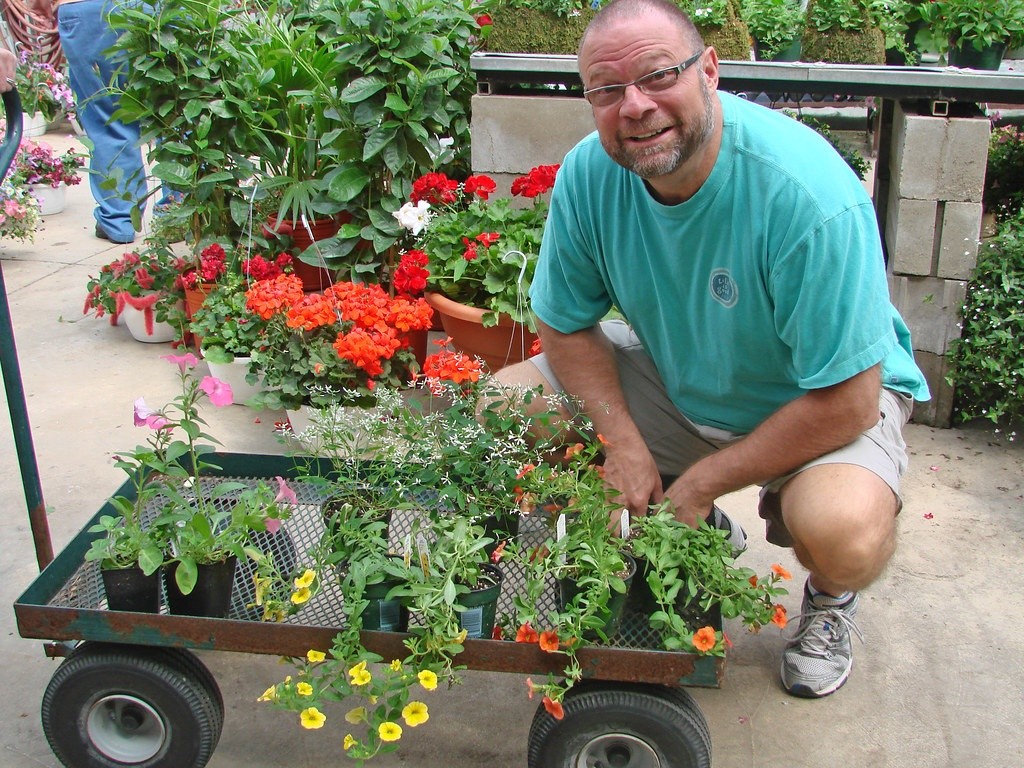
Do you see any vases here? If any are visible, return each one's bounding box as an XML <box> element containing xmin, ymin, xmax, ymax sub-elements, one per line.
<box><xmin>947</xmin><ymin>29</ymin><xmax>1011</xmax><ymax>71</ymax></box>
<box><xmin>22</xmin><ymin>109</ymin><xmax>48</xmax><ymax>139</ymax></box>
<box><xmin>557</xmin><ymin>547</ymin><xmax>636</xmax><ymax>640</ymax></box>
<box><xmin>753</xmin><ymin>29</ymin><xmax>800</xmax><ymax>62</ymax></box>
<box><xmin>277</xmin><ymin>396</ymin><xmax>384</xmax><ymax>459</ymax></box>
<box><xmin>432</xmin><ymin>561</ymin><xmax>505</xmax><ymax>638</ymax></box>
<box><xmin>420</xmin><ymin>289</ymin><xmax>543</xmax><ymax>375</ymax></box>
<box><xmin>118</xmin><ymin>291</ymin><xmax>186</xmax><ymax>342</ymax></box>
<box><xmin>341</xmin><ymin>551</ymin><xmax>419</xmax><ymax>632</ymax></box>
<box><xmin>885</xmin><ymin>18</ymin><xmax>929</xmax><ymax>66</ymax></box>
<box><xmin>100</xmin><ymin>555</ymin><xmax>163</xmax><ymax>613</ymax></box>
<box><xmin>619</xmin><ymin>528</ymin><xmax>699</xmax><ymax>614</ymax></box>
<box><xmin>166</xmin><ymin>559</ymin><xmax>238</xmax><ymax>616</ymax></box>
<box><xmin>19</xmin><ymin>181</ymin><xmax>68</xmax><ymax>217</ymax></box>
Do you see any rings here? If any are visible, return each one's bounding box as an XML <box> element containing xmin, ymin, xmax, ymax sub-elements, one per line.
<box><xmin>7</xmin><ymin>77</ymin><xmax>15</xmax><ymax>86</ymax></box>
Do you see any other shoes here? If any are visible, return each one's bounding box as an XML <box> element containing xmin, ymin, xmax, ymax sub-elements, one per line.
<box><xmin>95</xmin><ymin>222</ymin><xmax>119</xmax><ymax>243</ymax></box>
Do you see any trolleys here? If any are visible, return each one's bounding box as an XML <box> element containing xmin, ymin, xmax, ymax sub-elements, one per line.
<box><xmin>0</xmin><ymin>261</ymin><xmax>727</xmax><ymax>768</ymax></box>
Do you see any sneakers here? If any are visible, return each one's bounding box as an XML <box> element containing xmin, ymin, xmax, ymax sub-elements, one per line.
<box><xmin>779</xmin><ymin>577</ymin><xmax>865</xmax><ymax>698</ymax></box>
<box><xmin>698</xmin><ymin>503</ymin><xmax>747</xmax><ymax>562</ymax></box>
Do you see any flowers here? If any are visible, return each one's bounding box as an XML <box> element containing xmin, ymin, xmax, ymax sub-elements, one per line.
<box><xmin>161</xmin><ymin>352</ymin><xmax>300</xmax><ymax>597</ymax></box>
<box><xmin>634</xmin><ymin>498</ymin><xmax>797</xmax><ymax>658</ymax></box>
<box><xmin>185</xmin><ymin>243</ymin><xmax>293</xmax><ymax>287</ymax></box>
<box><xmin>83</xmin><ymin>249</ymin><xmax>193</xmax><ymax>336</ymax></box>
<box><xmin>269</xmin><ymin>276</ymin><xmax>435</xmax><ymax>408</ymax></box>
<box><xmin>392</xmin><ymin>162</ymin><xmax>562</xmax><ymax>321</ymax></box>
<box><xmin>988</xmin><ymin>110</ymin><xmax>1023</xmax><ymax>173</ymax></box>
<box><xmin>491</xmin><ymin>433</ymin><xmax>620</xmax><ymax>719</ymax></box>
<box><xmin>5</xmin><ymin>136</ymin><xmax>83</xmax><ymax>188</ymax></box>
<box><xmin>342</xmin><ymin>513</ymin><xmax>491</xmax><ymax>766</ymax></box>
<box><xmin>253</xmin><ymin>500</ymin><xmax>398</xmax><ymax>727</ymax></box>
<box><xmin>13</xmin><ymin>42</ymin><xmax>74</xmax><ymax>123</ymax></box>
<box><xmin>1</xmin><ymin>187</ymin><xmax>44</xmax><ymax>242</ymax></box>
<box><xmin>85</xmin><ymin>395</ymin><xmax>181</xmax><ymax>576</ymax></box>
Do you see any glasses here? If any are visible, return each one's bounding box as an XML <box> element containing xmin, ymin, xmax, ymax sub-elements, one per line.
<box><xmin>584</xmin><ymin>49</ymin><xmax>705</xmax><ymax>107</ymax></box>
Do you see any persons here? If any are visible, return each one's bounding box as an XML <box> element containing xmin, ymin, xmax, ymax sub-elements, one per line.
<box><xmin>0</xmin><ymin>48</ymin><xmax>20</xmax><ymax>93</ymax></box>
<box><xmin>57</xmin><ymin>0</ymin><xmax>205</xmax><ymax>245</ymax></box>
<box><xmin>476</xmin><ymin>0</ymin><xmax>932</xmax><ymax>701</ymax></box>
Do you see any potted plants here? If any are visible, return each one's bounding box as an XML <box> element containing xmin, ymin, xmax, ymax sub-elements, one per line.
<box><xmin>188</xmin><ymin>271</ymin><xmax>280</xmax><ymax>407</ymax></box>
<box><xmin>235</xmin><ymin>2</ymin><xmax>351</xmax><ymax>292</ymax></box>
<box><xmin>429</xmin><ymin>360</ymin><xmax>574</xmax><ymax>561</ymax></box>
<box><xmin>870</xmin><ymin>0</ymin><xmax>929</xmax><ymax>66</ymax></box>
<box><xmin>68</xmin><ymin>2</ymin><xmax>279</xmax><ymax>359</ymax></box>
<box><xmin>937</xmin><ymin>1</ymin><xmax>1024</xmax><ymax>71</ymax></box>
<box><xmin>739</xmin><ymin>0</ymin><xmax>802</xmax><ymax>63</ymax></box>
<box><xmin>274</xmin><ymin>380</ymin><xmax>440</xmax><ymax>561</ymax></box>
